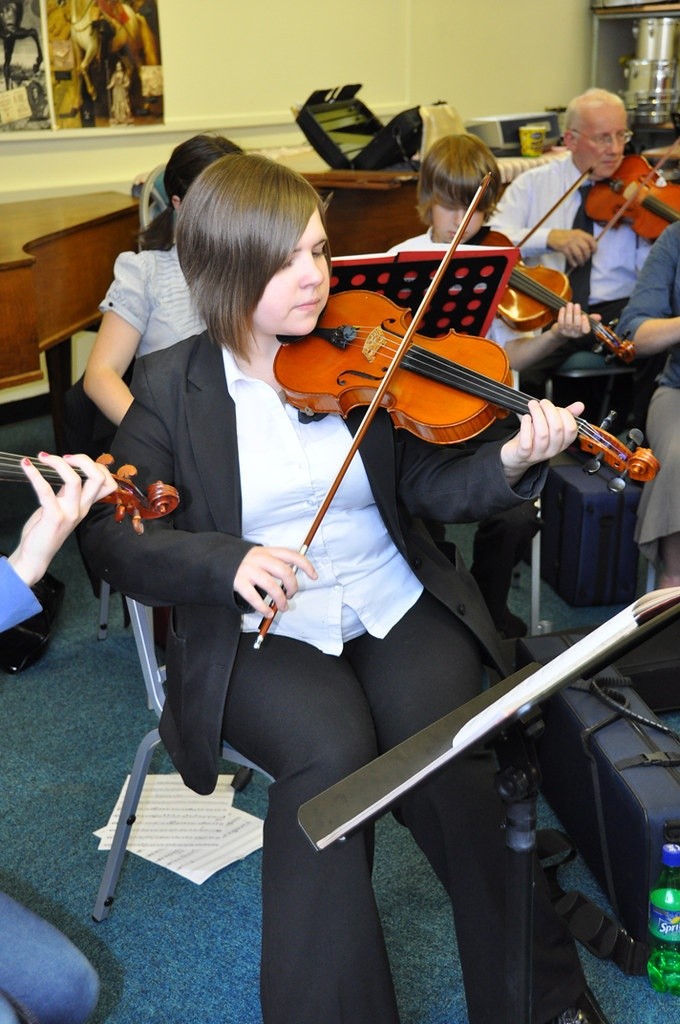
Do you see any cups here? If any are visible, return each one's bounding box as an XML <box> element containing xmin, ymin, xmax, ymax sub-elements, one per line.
<box><xmin>518</xmin><ymin>126</ymin><xmax>546</xmax><ymax>157</ymax></box>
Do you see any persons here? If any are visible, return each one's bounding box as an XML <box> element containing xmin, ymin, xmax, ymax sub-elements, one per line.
<box><xmin>0</xmin><ymin>451</ymin><xmax>118</xmax><ymax>1024</ymax></box>
<box><xmin>486</xmin><ymin>88</ymin><xmax>668</xmax><ymax>442</ymax></box>
<box><xmin>387</xmin><ymin>132</ymin><xmax>602</xmax><ymax>390</ymax></box>
<box><xmin>83</xmin><ymin>136</ymin><xmax>247</xmax><ymax>431</ymax></box>
<box><xmin>74</xmin><ymin>154</ymin><xmax>587</xmax><ymax>1024</ymax></box>
<box><xmin>612</xmin><ymin>221</ymin><xmax>680</xmax><ymax>592</ymax></box>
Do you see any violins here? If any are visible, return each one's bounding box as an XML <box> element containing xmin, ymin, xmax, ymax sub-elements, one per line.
<box><xmin>583</xmin><ymin>154</ymin><xmax>680</xmax><ymax>245</ymax></box>
<box><xmin>0</xmin><ymin>449</ymin><xmax>180</xmax><ymax>534</ymax></box>
<box><xmin>273</xmin><ymin>290</ymin><xmax>663</xmax><ymax>494</ymax></box>
<box><xmin>470</xmin><ymin>232</ymin><xmax>636</xmax><ymax>365</ymax></box>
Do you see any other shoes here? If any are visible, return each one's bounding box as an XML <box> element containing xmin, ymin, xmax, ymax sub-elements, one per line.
<box><xmin>486</xmin><ymin>602</ymin><xmax>529</xmax><ymax>637</ymax></box>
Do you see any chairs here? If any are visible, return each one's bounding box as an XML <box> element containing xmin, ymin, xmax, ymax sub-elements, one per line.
<box><xmin>58</xmin><ymin>352</ymin><xmax>656</xmax><ymax>922</ymax></box>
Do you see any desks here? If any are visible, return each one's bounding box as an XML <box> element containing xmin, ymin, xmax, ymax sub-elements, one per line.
<box><xmin>275</xmin><ymin>147</ymin><xmax>575</xmax><ymax>258</ymax></box>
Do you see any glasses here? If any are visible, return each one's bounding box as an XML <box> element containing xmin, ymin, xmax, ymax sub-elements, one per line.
<box><xmin>572</xmin><ymin>127</ymin><xmax>634</xmax><ymax>148</ymax></box>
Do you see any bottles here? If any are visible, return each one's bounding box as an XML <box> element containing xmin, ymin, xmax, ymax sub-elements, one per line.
<box><xmin>647</xmin><ymin>843</ymin><xmax>680</xmax><ymax>997</ymax></box>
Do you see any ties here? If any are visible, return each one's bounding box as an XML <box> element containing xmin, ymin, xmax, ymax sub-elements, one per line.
<box><xmin>565</xmin><ymin>184</ymin><xmax>595</xmax><ymax>309</ymax></box>
